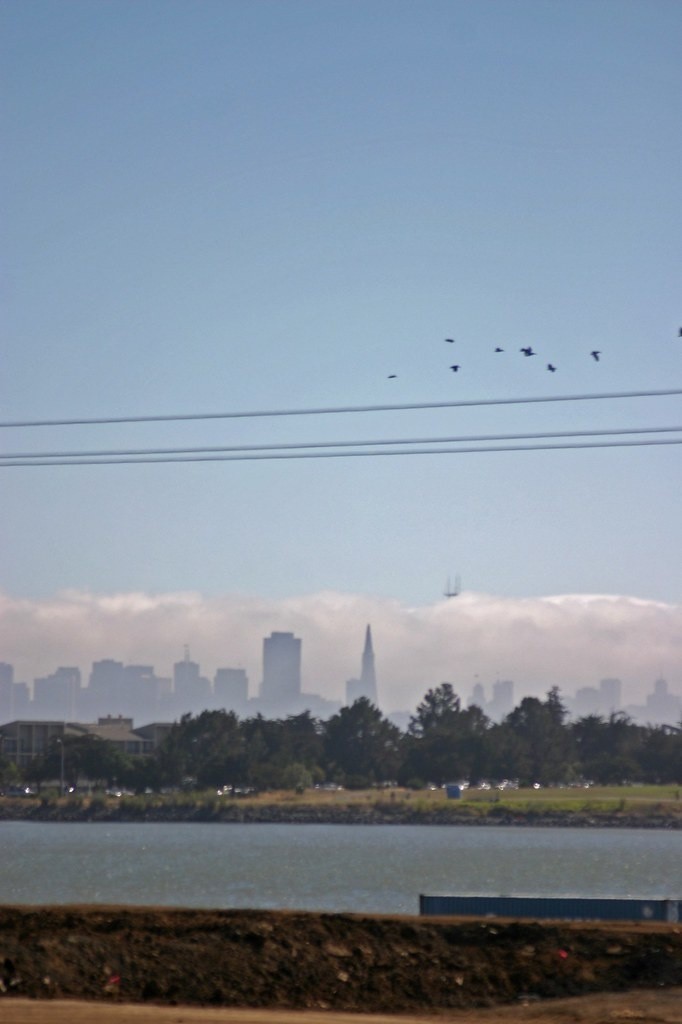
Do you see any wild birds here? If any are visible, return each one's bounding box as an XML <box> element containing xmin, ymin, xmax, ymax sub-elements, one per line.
<box><xmin>386</xmin><ymin>338</ymin><xmax>602</xmax><ymax>380</ymax></box>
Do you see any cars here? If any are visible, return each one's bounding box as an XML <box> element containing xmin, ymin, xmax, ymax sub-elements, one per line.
<box><xmin>106</xmin><ymin>786</ymin><xmax>134</xmax><ymax>797</ymax></box>
<box><xmin>315</xmin><ymin>783</ymin><xmax>344</xmax><ymax>792</ymax></box>
<box><xmin>374</xmin><ymin>780</ymin><xmax>397</xmax><ymax>788</ymax></box>
<box><xmin>425</xmin><ymin>779</ymin><xmax>592</xmax><ymax>789</ymax></box>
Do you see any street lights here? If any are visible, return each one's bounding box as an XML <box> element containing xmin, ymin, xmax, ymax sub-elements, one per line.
<box><xmin>56</xmin><ymin>739</ymin><xmax>64</xmax><ymax>800</ymax></box>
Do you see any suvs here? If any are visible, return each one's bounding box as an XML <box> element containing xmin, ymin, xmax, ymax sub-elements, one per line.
<box><xmin>5</xmin><ymin>787</ymin><xmax>37</xmax><ymax>798</ymax></box>
